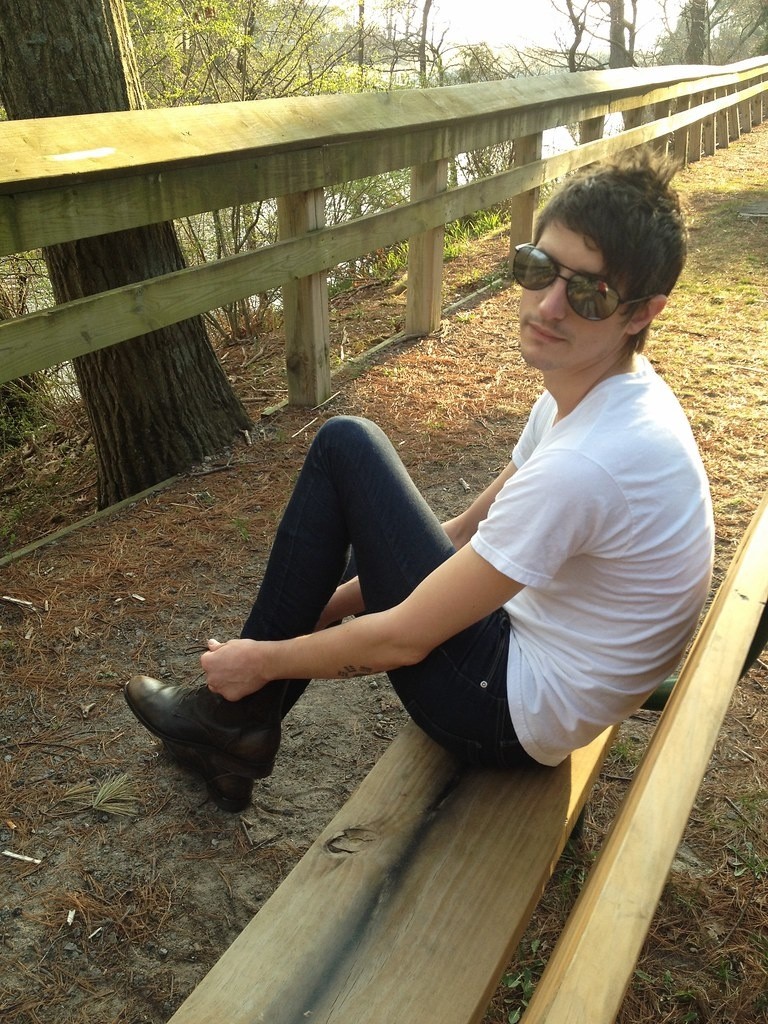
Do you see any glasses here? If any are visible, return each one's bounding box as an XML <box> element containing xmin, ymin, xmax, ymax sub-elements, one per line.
<box><xmin>512</xmin><ymin>243</ymin><xmax>656</xmax><ymax>321</ymax></box>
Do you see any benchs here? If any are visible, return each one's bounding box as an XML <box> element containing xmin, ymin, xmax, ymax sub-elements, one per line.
<box><xmin>166</xmin><ymin>488</ymin><xmax>768</xmax><ymax>1023</ymax></box>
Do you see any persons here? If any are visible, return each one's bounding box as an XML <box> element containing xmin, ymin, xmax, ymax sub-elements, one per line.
<box><xmin>123</xmin><ymin>135</ymin><xmax>715</xmax><ymax>812</ymax></box>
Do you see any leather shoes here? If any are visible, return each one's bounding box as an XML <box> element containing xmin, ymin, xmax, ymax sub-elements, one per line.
<box><xmin>162</xmin><ymin>738</ymin><xmax>255</xmax><ymax>811</ymax></box>
<box><xmin>123</xmin><ymin>646</ymin><xmax>281</xmax><ymax>779</ymax></box>
